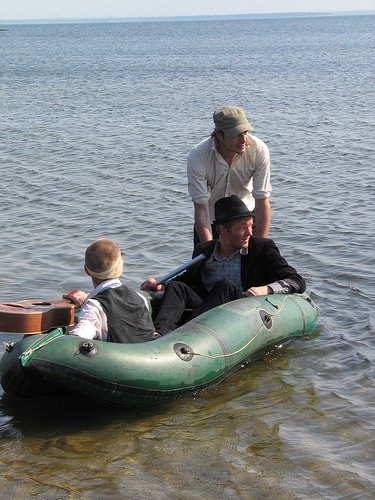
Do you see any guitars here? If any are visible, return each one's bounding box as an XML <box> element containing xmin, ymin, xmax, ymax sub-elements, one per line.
<box><xmin>0</xmin><ymin>299</ymin><xmax>76</xmax><ymax>334</ymax></box>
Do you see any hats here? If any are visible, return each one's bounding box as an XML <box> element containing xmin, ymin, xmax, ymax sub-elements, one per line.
<box><xmin>213</xmin><ymin>105</ymin><xmax>252</xmax><ymax>138</ymax></box>
<box><xmin>213</xmin><ymin>195</ymin><xmax>255</xmax><ymax>223</ymax></box>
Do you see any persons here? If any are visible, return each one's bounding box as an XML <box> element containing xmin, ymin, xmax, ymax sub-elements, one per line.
<box><xmin>139</xmin><ymin>194</ymin><xmax>306</xmax><ymax>340</ymax></box>
<box><xmin>63</xmin><ymin>237</ymin><xmax>162</xmax><ymax>343</ymax></box>
<box><xmin>187</xmin><ymin>106</ymin><xmax>273</xmax><ymax>259</ymax></box>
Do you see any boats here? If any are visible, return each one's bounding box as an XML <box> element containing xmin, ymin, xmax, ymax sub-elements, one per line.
<box><xmin>0</xmin><ymin>293</ymin><xmax>322</xmax><ymax>407</ymax></box>
